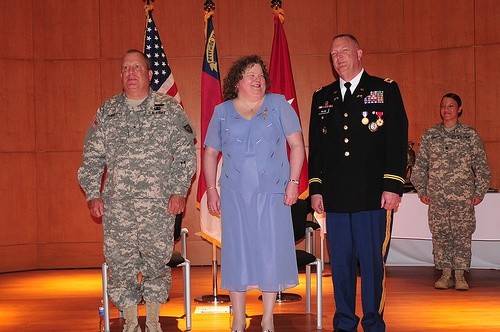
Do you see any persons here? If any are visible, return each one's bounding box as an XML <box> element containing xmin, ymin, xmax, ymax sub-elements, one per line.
<box><xmin>201</xmin><ymin>56</ymin><xmax>306</xmax><ymax>332</ymax></box>
<box><xmin>410</xmin><ymin>94</ymin><xmax>491</xmax><ymax>290</ymax></box>
<box><xmin>308</xmin><ymin>34</ymin><xmax>409</xmax><ymax>332</ymax></box>
<box><xmin>79</xmin><ymin>50</ymin><xmax>198</xmax><ymax>332</ymax></box>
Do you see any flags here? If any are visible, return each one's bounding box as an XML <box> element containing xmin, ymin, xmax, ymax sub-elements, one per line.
<box><xmin>144</xmin><ymin>4</ymin><xmax>310</xmax><ymax>252</ymax></box>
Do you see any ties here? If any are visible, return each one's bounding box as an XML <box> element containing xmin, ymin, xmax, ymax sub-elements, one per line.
<box><xmin>343</xmin><ymin>81</ymin><xmax>355</xmax><ymax>100</ymax></box>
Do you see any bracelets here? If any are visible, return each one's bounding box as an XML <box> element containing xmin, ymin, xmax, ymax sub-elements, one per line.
<box><xmin>290</xmin><ymin>179</ymin><xmax>299</xmax><ymax>184</ymax></box>
<box><xmin>207</xmin><ymin>186</ymin><xmax>216</xmax><ymax>190</ymax></box>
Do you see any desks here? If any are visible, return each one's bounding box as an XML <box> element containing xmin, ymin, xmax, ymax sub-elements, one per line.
<box><xmin>384</xmin><ymin>192</ymin><xmax>500</xmax><ymax>270</ymax></box>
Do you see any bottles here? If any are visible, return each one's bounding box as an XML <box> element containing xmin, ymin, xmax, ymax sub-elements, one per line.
<box><xmin>99</xmin><ymin>299</ymin><xmax>104</xmax><ymax>332</ymax></box>
<box><xmin>229</xmin><ymin>303</ymin><xmax>233</xmax><ymax>329</ymax></box>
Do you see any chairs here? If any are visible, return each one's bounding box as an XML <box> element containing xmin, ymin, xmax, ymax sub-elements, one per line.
<box><xmin>290</xmin><ymin>197</ymin><xmax>323</xmax><ymax>331</ymax></box>
<box><xmin>101</xmin><ymin>211</ymin><xmax>192</xmax><ymax>332</ymax></box>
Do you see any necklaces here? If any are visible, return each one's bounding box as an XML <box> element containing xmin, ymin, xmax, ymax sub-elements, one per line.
<box><xmin>241</xmin><ymin>96</ymin><xmax>260</xmax><ymax>112</ymax></box>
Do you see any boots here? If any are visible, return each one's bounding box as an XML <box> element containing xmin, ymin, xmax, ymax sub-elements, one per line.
<box><xmin>122</xmin><ymin>306</ymin><xmax>142</xmax><ymax>332</ymax></box>
<box><xmin>455</xmin><ymin>270</ymin><xmax>468</xmax><ymax>290</ymax></box>
<box><xmin>145</xmin><ymin>302</ymin><xmax>163</xmax><ymax>332</ymax></box>
<box><xmin>435</xmin><ymin>267</ymin><xmax>453</xmax><ymax>289</ymax></box>
